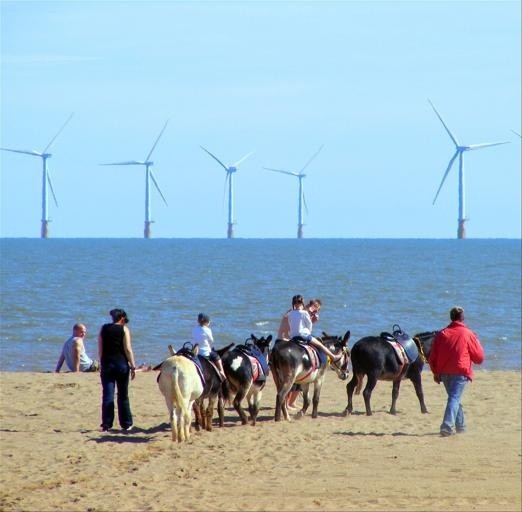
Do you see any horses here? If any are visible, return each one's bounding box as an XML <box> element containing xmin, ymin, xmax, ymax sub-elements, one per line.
<box><xmin>344</xmin><ymin>331</ymin><xmax>439</xmax><ymax>416</ymax></box>
<box><xmin>158</xmin><ymin>334</ymin><xmax>272</xmax><ymax>443</ymax></box>
<box><xmin>268</xmin><ymin>331</ymin><xmax>351</xmax><ymax>422</ymax></box>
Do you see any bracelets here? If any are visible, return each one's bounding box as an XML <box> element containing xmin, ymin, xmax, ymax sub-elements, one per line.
<box><xmin>130</xmin><ymin>367</ymin><xmax>135</xmax><ymax>370</ymax></box>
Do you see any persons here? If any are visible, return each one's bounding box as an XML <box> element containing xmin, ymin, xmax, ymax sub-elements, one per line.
<box><xmin>277</xmin><ymin>299</ymin><xmax>321</xmax><ymax>407</ymax></box>
<box><xmin>55</xmin><ymin>322</ymin><xmax>99</xmax><ymax>373</ymax></box>
<box><xmin>97</xmin><ymin>309</ymin><xmax>136</xmax><ymax>433</ymax></box>
<box><xmin>429</xmin><ymin>306</ymin><xmax>485</xmax><ymax>437</ymax></box>
<box><xmin>191</xmin><ymin>312</ymin><xmax>227</xmax><ymax>379</ymax></box>
<box><xmin>286</xmin><ymin>294</ymin><xmax>343</xmax><ymax>363</ymax></box>
